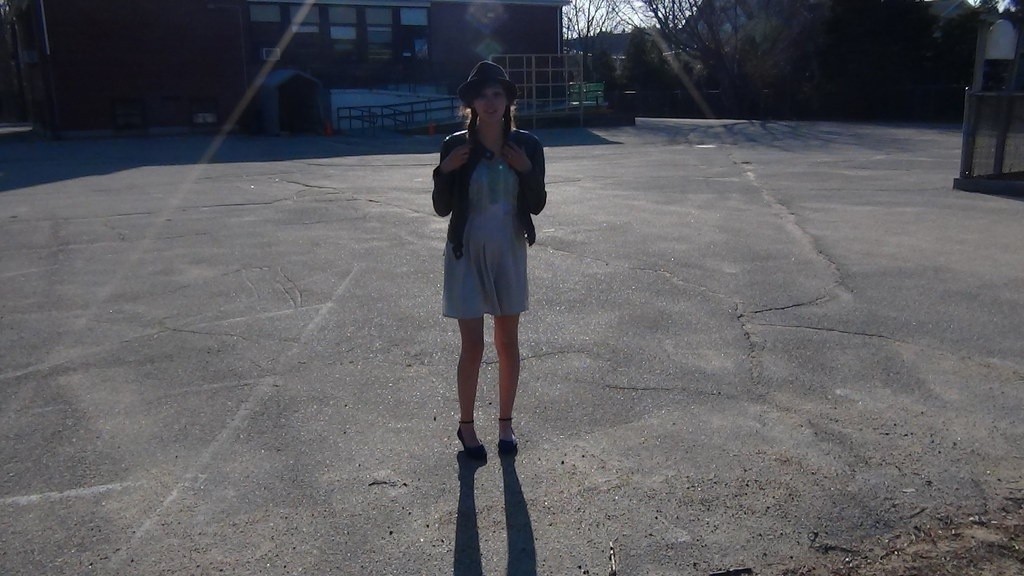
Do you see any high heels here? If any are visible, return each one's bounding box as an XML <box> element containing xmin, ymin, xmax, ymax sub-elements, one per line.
<box><xmin>498</xmin><ymin>418</ymin><xmax>517</xmax><ymax>455</ymax></box>
<box><xmin>456</xmin><ymin>419</ymin><xmax>487</xmax><ymax>459</ymax></box>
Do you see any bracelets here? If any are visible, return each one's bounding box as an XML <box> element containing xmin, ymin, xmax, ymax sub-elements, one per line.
<box><xmin>526</xmin><ymin>161</ymin><xmax>531</xmax><ymax>173</ymax></box>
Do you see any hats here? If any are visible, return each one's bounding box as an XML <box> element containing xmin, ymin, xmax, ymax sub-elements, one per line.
<box><xmin>458</xmin><ymin>61</ymin><xmax>517</xmax><ymax>108</ymax></box>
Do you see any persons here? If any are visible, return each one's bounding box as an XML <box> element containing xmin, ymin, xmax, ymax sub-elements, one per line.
<box><xmin>431</xmin><ymin>62</ymin><xmax>547</xmax><ymax>460</ymax></box>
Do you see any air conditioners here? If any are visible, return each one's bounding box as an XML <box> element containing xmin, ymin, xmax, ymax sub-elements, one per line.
<box><xmin>260</xmin><ymin>47</ymin><xmax>281</xmax><ymax>62</ymax></box>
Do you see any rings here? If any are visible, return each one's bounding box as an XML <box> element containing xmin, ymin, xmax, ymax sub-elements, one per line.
<box><xmin>507</xmin><ymin>155</ymin><xmax>512</xmax><ymax>159</ymax></box>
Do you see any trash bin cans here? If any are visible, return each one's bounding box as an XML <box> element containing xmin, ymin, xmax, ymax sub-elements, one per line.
<box><xmin>244</xmin><ymin>64</ymin><xmax>306</xmax><ymax>134</ymax></box>
